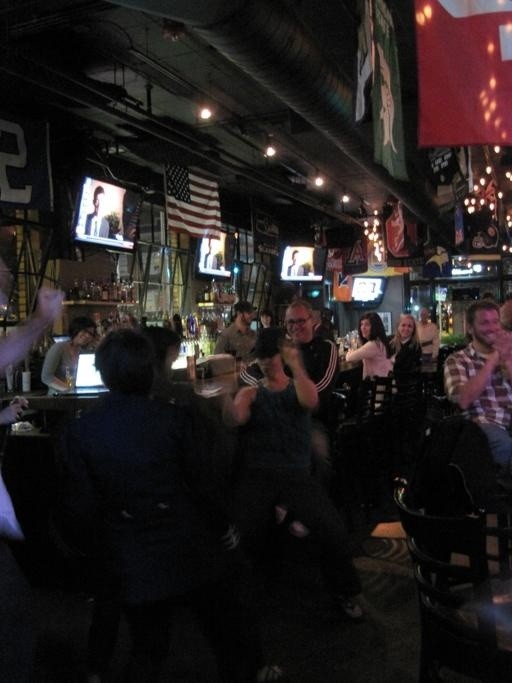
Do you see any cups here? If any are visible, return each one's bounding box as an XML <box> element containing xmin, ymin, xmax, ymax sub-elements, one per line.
<box><xmin>6</xmin><ymin>364</ymin><xmax>32</xmax><ymax>393</ymax></box>
<box><xmin>66</xmin><ymin>367</ymin><xmax>76</xmax><ymax>393</ymax></box>
<box><xmin>336</xmin><ymin>330</ymin><xmax>359</xmax><ymax>362</ymax></box>
<box><xmin>187</xmin><ymin>356</ymin><xmax>196</xmax><ymax>380</ymax></box>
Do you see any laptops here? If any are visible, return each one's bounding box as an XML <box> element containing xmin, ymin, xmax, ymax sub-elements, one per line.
<box><xmin>54</xmin><ymin>354</ymin><xmax>111</xmax><ymax>395</ymax></box>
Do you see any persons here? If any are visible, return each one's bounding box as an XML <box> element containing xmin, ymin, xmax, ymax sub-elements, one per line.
<box><xmin>0</xmin><ymin>256</ymin><xmax>68</xmax><ymax>543</ymax></box>
<box><xmin>85</xmin><ymin>186</ymin><xmax>110</xmax><ymax>238</ymax></box>
<box><xmin>117</xmin><ymin>326</ymin><xmax>294</xmax><ymax>682</ymax></box>
<box><xmin>293</xmin><ymin>251</ymin><xmax>310</xmax><ymax>276</ymax></box>
<box><xmin>357</xmin><ymin>281</ymin><xmax>376</xmax><ymax>294</ymax></box>
<box><xmin>424</xmin><ymin>244</ymin><xmax>449</xmax><ymax>275</ymax></box>
<box><xmin>182</xmin><ymin>328</ymin><xmax>380</xmax><ymax>622</ymax></box>
<box><xmin>337</xmin><ymin>289</ymin><xmax>512</xmax><ymax>488</ymax></box>
<box><xmin>39</xmin><ymin>316</ymin><xmax>96</xmax><ymax>396</ymax></box>
<box><xmin>211</xmin><ymin>296</ymin><xmax>338</xmax><ymax>475</ymax></box>
<box><xmin>57</xmin><ymin>328</ymin><xmax>257</xmax><ymax>682</ymax></box>
<box><xmin>206</xmin><ymin>234</ymin><xmax>223</xmax><ymax>269</ymax></box>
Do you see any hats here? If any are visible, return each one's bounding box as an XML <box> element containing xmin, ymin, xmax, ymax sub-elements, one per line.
<box><xmin>251</xmin><ymin>326</ymin><xmax>281</xmax><ymax>358</ymax></box>
<box><xmin>234</xmin><ymin>301</ymin><xmax>257</xmax><ymax>311</ymax></box>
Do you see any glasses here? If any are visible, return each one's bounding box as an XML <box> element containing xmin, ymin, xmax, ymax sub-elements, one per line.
<box><xmin>287</xmin><ymin>316</ymin><xmax>310</xmax><ymax>324</ymax></box>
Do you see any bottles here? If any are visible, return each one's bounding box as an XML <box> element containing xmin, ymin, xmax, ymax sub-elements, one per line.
<box><xmin>69</xmin><ymin>272</ymin><xmax>235</xmax><ymax>358</ymax></box>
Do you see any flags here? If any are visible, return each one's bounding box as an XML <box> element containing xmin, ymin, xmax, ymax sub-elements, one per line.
<box><xmin>164</xmin><ymin>161</ymin><xmax>222</xmax><ymax>239</ymax></box>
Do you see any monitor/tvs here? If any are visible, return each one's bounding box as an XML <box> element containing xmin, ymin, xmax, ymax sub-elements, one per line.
<box><xmin>194</xmin><ymin>225</ymin><xmax>233</xmax><ymax>280</ymax></box>
<box><xmin>351</xmin><ymin>277</ymin><xmax>385</xmax><ymax>304</ymax></box>
<box><xmin>67</xmin><ymin>174</ymin><xmax>144</xmax><ymax>254</ymax></box>
<box><xmin>278</xmin><ymin>241</ymin><xmax>326</xmax><ymax>285</ymax></box>
